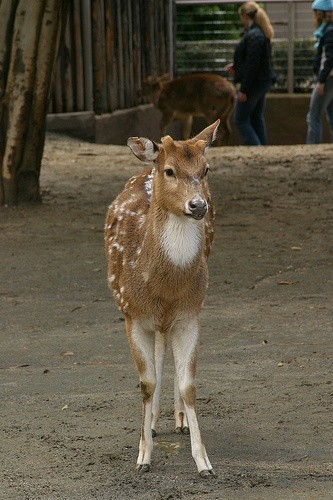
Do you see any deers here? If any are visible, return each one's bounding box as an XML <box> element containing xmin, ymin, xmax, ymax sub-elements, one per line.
<box><xmin>142</xmin><ymin>71</ymin><xmax>237</xmax><ymax>146</ymax></box>
<box><xmin>104</xmin><ymin>118</ymin><xmax>221</xmax><ymax>478</ymax></box>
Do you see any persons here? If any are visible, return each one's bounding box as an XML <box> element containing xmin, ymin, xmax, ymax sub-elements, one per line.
<box><xmin>307</xmin><ymin>0</ymin><xmax>332</xmax><ymax>143</ymax></box>
<box><xmin>226</xmin><ymin>0</ymin><xmax>276</xmax><ymax>146</ymax></box>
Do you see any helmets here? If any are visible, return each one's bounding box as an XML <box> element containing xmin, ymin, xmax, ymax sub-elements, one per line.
<box><xmin>311</xmin><ymin>0</ymin><xmax>333</xmax><ymax>11</ymax></box>
